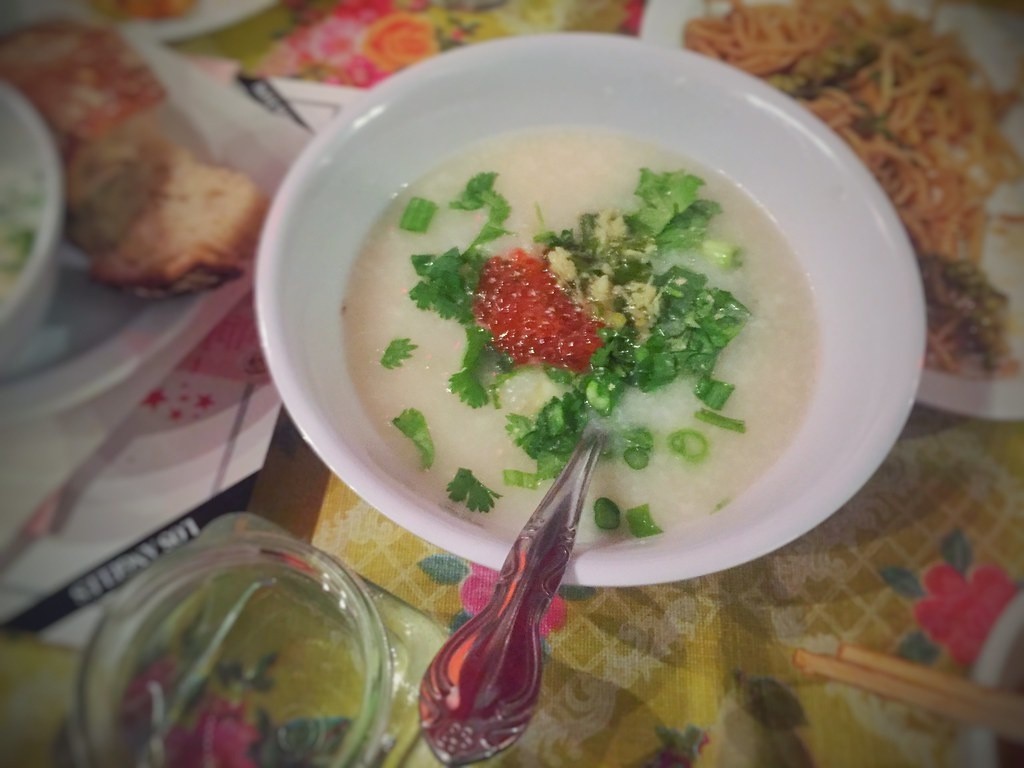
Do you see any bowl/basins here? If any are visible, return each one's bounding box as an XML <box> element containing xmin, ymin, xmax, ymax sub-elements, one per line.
<box><xmin>254</xmin><ymin>31</ymin><xmax>924</xmax><ymax>586</ymax></box>
<box><xmin>0</xmin><ymin>78</ymin><xmax>63</xmax><ymax>326</ymax></box>
<box><xmin>78</xmin><ymin>531</ymin><xmax>392</xmax><ymax>768</ymax></box>
<box><xmin>968</xmin><ymin>593</ymin><xmax>1024</xmax><ymax>768</ymax></box>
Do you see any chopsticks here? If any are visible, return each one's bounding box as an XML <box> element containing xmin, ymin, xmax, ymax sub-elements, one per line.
<box><xmin>792</xmin><ymin>645</ymin><xmax>1024</xmax><ymax>739</ymax></box>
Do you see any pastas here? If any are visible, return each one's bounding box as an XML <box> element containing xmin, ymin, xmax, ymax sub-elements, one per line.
<box><xmin>681</xmin><ymin>0</ymin><xmax>1024</xmax><ymax>380</ymax></box>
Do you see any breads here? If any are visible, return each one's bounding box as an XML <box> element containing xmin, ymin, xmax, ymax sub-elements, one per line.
<box><xmin>59</xmin><ymin>132</ymin><xmax>269</xmax><ymax>299</ymax></box>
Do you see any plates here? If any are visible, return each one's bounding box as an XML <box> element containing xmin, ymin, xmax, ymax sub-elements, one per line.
<box><xmin>634</xmin><ymin>0</ymin><xmax>1024</xmax><ymax>420</ymax></box>
<box><xmin>33</xmin><ymin>0</ymin><xmax>284</xmax><ymax>42</ymax></box>
<box><xmin>0</xmin><ymin>25</ymin><xmax>218</xmax><ymax>421</ymax></box>
<box><xmin>189</xmin><ymin>512</ymin><xmax>448</xmax><ymax>768</ymax></box>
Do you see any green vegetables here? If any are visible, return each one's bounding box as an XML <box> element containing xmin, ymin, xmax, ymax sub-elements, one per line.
<box><xmin>382</xmin><ymin>167</ymin><xmax>753</xmax><ymax>538</ymax></box>
